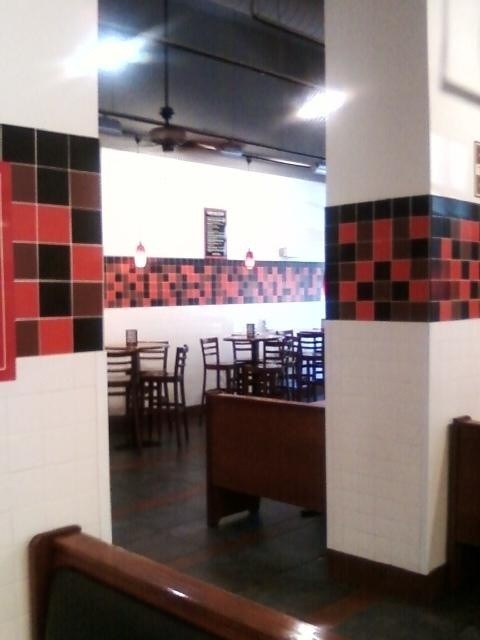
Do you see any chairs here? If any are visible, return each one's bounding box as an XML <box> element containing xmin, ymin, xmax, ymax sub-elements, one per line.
<box><xmin>197</xmin><ymin>385</ymin><xmax>326</xmax><ymax>531</ymax></box>
<box><xmin>104</xmin><ymin>323</ymin><xmax>326</xmax><ymax>456</ymax></box>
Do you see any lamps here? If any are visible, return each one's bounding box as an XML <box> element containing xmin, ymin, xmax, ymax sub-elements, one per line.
<box><xmin>147</xmin><ymin>1</ymin><xmax>188</xmax><ymax>155</ymax></box>
